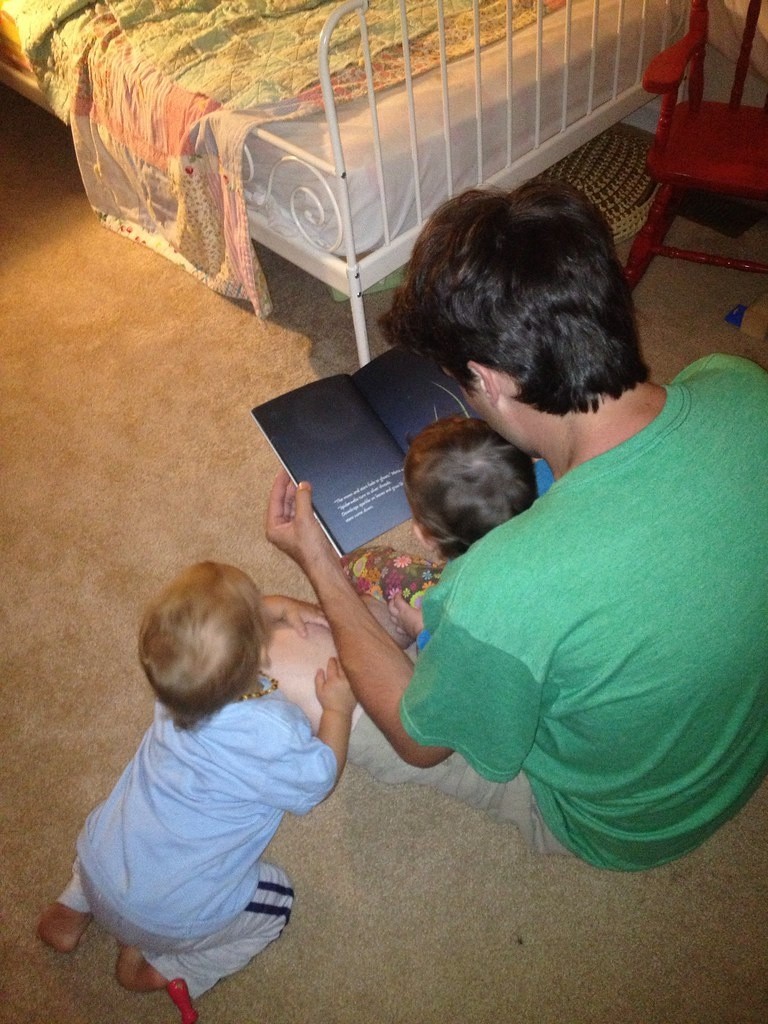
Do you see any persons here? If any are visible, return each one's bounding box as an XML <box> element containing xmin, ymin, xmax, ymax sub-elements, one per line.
<box><xmin>266</xmin><ymin>172</ymin><xmax>767</xmax><ymax>870</ymax></box>
<box><xmin>339</xmin><ymin>415</ymin><xmax>556</xmax><ymax>662</ymax></box>
<box><xmin>34</xmin><ymin>561</ymin><xmax>358</xmax><ymax>996</ymax></box>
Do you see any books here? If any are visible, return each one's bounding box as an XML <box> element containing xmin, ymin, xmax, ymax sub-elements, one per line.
<box><xmin>251</xmin><ymin>345</ymin><xmax>482</xmax><ymax>557</ymax></box>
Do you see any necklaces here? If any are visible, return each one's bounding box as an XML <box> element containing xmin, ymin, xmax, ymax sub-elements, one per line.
<box><xmin>233</xmin><ymin>670</ymin><xmax>280</xmax><ymax>701</ymax></box>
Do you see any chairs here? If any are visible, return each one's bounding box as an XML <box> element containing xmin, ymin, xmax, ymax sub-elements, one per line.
<box><xmin>621</xmin><ymin>0</ymin><xmax>768</xmax><ymax>294</ymax></box>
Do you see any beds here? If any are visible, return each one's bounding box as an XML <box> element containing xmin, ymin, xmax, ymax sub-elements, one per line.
<box><xmin>0</xmin><ymin>0</ymin><xmax>705</xmax><ymax>373</ymax></box>
